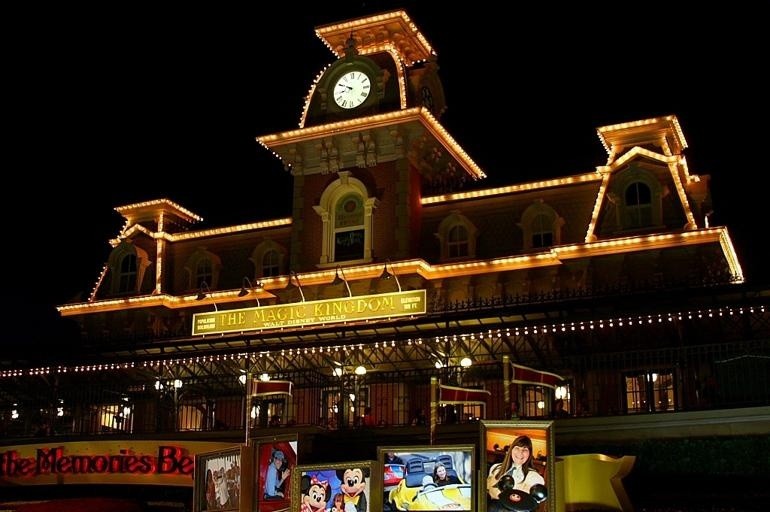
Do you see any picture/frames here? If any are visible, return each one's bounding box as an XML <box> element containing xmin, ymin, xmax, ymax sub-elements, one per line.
<box><xmin>194</xmin><ymin>418</ymin><xmax>556</xmax><ymax>512</ymax></box>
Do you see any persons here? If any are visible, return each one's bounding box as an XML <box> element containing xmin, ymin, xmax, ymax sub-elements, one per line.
<box><xmin>263</xmin><ymin>450</ymin><xmax>291</xmax><ymax>499</ymax></box>
<box><xmin>412</xmin><ymin>407</ymin><xmax>429</xmax><ymax>425</ymax></box>
<box><xmin>501</xmin><ymin>401</ymin><xmax>527</xmax><ymax>418</ymax></box>
<box><xmin>205</xmin><ymin>462</ymin><xmax>241</xmax><ymax>511</ymax></box>
<box><xmin>359</xmin><ymin>407</ymin><xmax>375</xmax><ymax>425</ymax></box>
<box><xmin>429</xmin><ymin>462</ymin><xmax>464</xmax><ymax>486</ymax></box>
<box><xmin>546</xmin><ymin>397</ymin><xmax>577</xmax><ymax>419</ymax></box>
<box><xmin>485</xmin><ymin>435</ymin><xmax>546</xmax><ymax>511</ymax></box>
<box><xmin>270</xmin><ymin>414</ymin><xmax>283</xmax><ymax>426</ymax></box>
<box><xmin>385</xmin><ymin>451</ymin><xmax>402</xmax><ymax>464</ymax></box>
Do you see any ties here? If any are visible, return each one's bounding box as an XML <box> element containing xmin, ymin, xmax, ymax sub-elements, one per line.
<box><xmin>492</xmin><ymin>467</ymin><xmax>517</xmax><ymax>490</ymax></box>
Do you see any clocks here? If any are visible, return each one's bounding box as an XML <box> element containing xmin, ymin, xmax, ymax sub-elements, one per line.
<box><xmin>333</xmin><ymin>70</ymin><xmax>371</xmax><ymax>110</ymax></box>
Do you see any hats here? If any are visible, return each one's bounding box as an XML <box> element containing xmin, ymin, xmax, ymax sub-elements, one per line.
<box><xmin>272</xmin><ymin>450</ymin><xmax>285</xmax><ymax>459</ymax></box>
<box><xmin>422</xmin><ymin>475</ymin><xmax>434</xmax><ymax>485</ymax></box>
